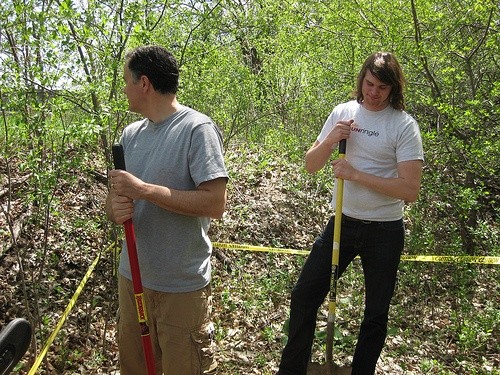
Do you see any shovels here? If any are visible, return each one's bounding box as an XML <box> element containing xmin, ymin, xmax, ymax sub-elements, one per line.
<box><xmin>306</xmin><ymin>138</ymin><xmax>353</xmax><ymax>375</ymax></box>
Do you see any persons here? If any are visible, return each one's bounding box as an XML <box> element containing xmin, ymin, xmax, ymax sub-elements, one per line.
<box><xmin>278</xmin><ymin>48</ymin><xmax>425</xmax><ymax>375</ymax></box>
<box><xmin>104</xmin><ymin>47</ymin><xmax>229</xmax><ymax>375</ymax></box>
